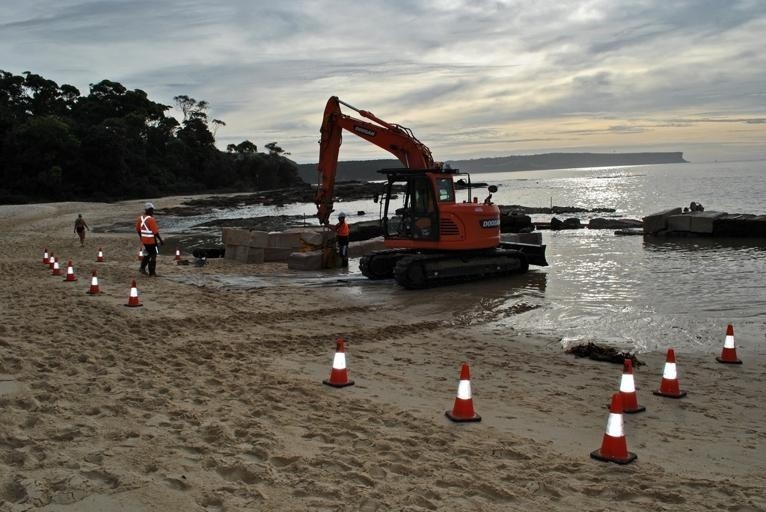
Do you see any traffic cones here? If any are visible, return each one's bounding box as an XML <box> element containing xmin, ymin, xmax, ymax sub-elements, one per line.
<box><xmin>124</xmin><ymin>280</ymin><xmax>142</xmax><ymax>308</ymax></box>
<box><xmin>714</xmin><ymin>323</ymin><xmax>742</xmax><ymax>365</ymax></box>
<box><xmin>173</xmin><ymin>247</ymin><xmax>180</xmax><ymax>259</ymax></box>
<box><xmin>95</xmin><ymin>248</ymin><xmax>104</xmax><ymax>263</ymax></box>
<box><xmin>85</xmin><ymin>271</ymin><xmax>101</xmax><ymax>295</ymax></box>
<box><xmin>40</xmin><ymin>248</ymin><xmax>48</xmax><ymax>264</ymax></box>
<box><xmin>443</xmin><ymin>364</ymin><xmax>482</xmax><ymax>424</ymax></box>
<box><xmin>322</xmin><ymin>340</ymin><xmax>354</xmax><ymax>389</ymax></box>
<box><xmin>50</xmin><ymin>257</ymin><xmax>63</xmax><ymax>275</ymax></box>
<box><xmin>62</xmin><ymin>258</ymin><xmax>77</xmax><ymax>281</ymax></box>
<box><xmin>608</xmin><ymin>360</ymin><xmax>645</xmax><ymax>414</ymax></box>
<box><xmin>590</xmin><ymin>393</ymin><xmax>636</xmax><ymax>466</ymax></box>
<box><xmin>653</xmin><ymin>348</ymin><xmax>687</xmax><ymax>399</ymax></box>
<box><xmin>47</xmin><ymin>253</ymin><xmax>54</xmax><ymax>269</ymax></box>
<box><xmin>136</xmin><ymin>247</ymin><xmax>144</xmax><ymax>261</ymax></box>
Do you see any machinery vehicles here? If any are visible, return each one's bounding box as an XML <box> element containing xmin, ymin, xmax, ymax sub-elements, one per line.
<box><xmin>315</xmin><ymin>95</ymin><xmax>548</xmax><ymax>290</ymax></box>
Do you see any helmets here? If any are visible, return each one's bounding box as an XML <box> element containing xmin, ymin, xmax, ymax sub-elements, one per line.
<box><xmin>144</xmin><ymin>202</ymin><xmax>156</xmax><ymax>209</ymax></box>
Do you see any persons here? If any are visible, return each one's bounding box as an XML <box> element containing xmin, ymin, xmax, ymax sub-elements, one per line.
<box><xmin>324</xmin><ymin>211</ymin><xmax>351</xmax><ymax>269</ymax></box>
<box><xmin>136</xmin><ymin>202</ymin><xmax>165</xmax><ymax>277</ymax></box>
<box><xmin>73</xmin><ymin>213</ymin><xmax>90</xmax><ymax>248</ymax></box>
